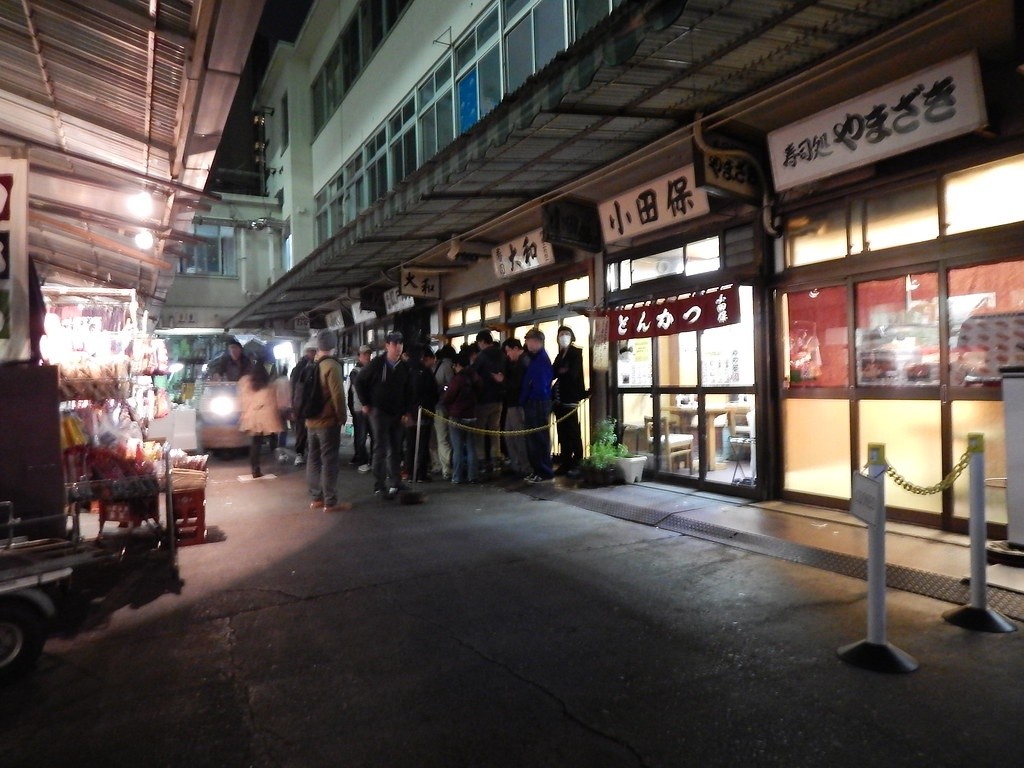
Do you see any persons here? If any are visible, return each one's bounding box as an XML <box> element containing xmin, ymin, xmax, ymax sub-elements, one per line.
<box><xmin>204</xmin><ymin>326</ymin><xmax>584</xmax><ymax>513</ymax></box>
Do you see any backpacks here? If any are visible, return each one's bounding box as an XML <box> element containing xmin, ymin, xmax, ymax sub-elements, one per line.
<box><xmin>294</xmin><ymin>355</ymin><xmax>344</xmax><ymax>419</ymax></box>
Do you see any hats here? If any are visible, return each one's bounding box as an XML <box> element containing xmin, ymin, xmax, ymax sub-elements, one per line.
<box><xmin>524</xmin><ymin>329</ymin><xmax>546</xmax><ymax>341</ymax></box>
<box><xmin>385</xmin><ymin>331</ymin><xmax>405</xmax><ymax>342</ymax></box>
<box><xmin>557</xmin><ymin>326</ymin><xmax>575</xmax><ymax>341</ymax></box>
<box><xmin>359</xmin><ymin>345</ymin><xmax>371</xmax><ymax>353</ymax></box>
<box><xmin>316</xmin><ymin>329</ymin><xmax>337</xmax><ymax>351</ymax></box>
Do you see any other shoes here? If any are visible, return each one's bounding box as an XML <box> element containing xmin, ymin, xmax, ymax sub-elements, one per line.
<box><xmin>309</xmin><ymin>500</ymin><xmax>324</xmax><ymax>509</ymax></box>
<box><xmin>323</xmin><ymin>501</ymin><xmax>353</xmax><ymax>513</ymax></box>
<box><xmin>529</xmin><ymin>475</ymin><xmax>554</xmax><ymax>484</ymax></box>
<box><xmin>397</xmin><ymin>452</ymin><xmax>513</xmax><ymax>486</ymax></box>
<box><xmin>294</xmin><ymin>453</ymin><xmax>305</xmax><ymax>467</ymax></box>
<box><xmin>387</xmin><ymin>487</ymin><xmax>400</xmax><ymax>498</ymax></box>
<box><xmin>553</xmin><ymin>462</ymin><xmax>571</xmax><ymax>475</ymax></box>
<box><xmin>357</xmin><ymin>464</ymin><xmax>370</xmax><ymax>472</ymax></box>
<box><xmin>280</xmin><ymin>454</ymin><xmax>289</xmax><ymax>464</ymax></box>
<box><xmin>524</xmin><ymin>472</ymin><xmax>535</xmax><ymax>481</ymax></box>
<box><xmin>566</xmin><ymin>465</ymin><xmax>584</xmax><ymax>477</ymax></box>
<box><xmin>253</xmin><ymin>471</ymin><xmax>263</xmax><ymax>478</ymax></box>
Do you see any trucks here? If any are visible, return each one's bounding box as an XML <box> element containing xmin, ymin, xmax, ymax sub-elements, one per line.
<box><xmin>0</xmin><ymin>501</ymin><xmax>121</xmax><ymax>692</ymax></box>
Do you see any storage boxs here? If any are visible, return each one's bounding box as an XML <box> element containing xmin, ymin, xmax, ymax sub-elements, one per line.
<box><xmin>98</xmin><ymin>492</ymin><xmax>160</xmax><ymax>537</ymax></box>
<box><xmin>166</xmin><ymin>489</ymin><xmax>205</xmax><ymax>547</ymax></box>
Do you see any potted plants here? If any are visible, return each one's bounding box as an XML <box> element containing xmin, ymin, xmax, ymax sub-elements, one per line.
<box><xmin>577</xmin><ymin>416</ymin><xmax>629</xmax><ymax>488</ymax></box>
<box><xmin>615</xmin><ymin>441</ymin><xmax>648</xmax><ymax>484</ymax></box>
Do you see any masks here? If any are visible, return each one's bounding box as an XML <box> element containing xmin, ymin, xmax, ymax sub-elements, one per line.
<box><xmin>559</xmin><ymin>334</ymin><xmax>572</xmax><ymax>348</ymax></box>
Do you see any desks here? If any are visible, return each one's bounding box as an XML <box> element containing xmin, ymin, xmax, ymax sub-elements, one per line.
<box><xmin>660</xmin><ymin>406</ymin><xmax>727</xmax><ymax>471</ymax></box>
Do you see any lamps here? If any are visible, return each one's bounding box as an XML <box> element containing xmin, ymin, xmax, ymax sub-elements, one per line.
<box><xmin>128</xmin><ymin>184</ymin><xmax>155</xmax><ymax>218</ymax></box>
<box><xmin>134</xmin><ymin>224</ymin><xmax>154</xmax><ymax>250</ymax></box>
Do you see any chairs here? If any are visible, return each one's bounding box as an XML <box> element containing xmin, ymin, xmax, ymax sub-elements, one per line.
<box><xmin>646</xmin><ymin>417</ymin><xmax>694</xmax><ymax>475</ymax></box>
<box><xmin>729</xmin><ymin>407</ymin><xmax>751</xmax><ymax>461</ymax></box>
